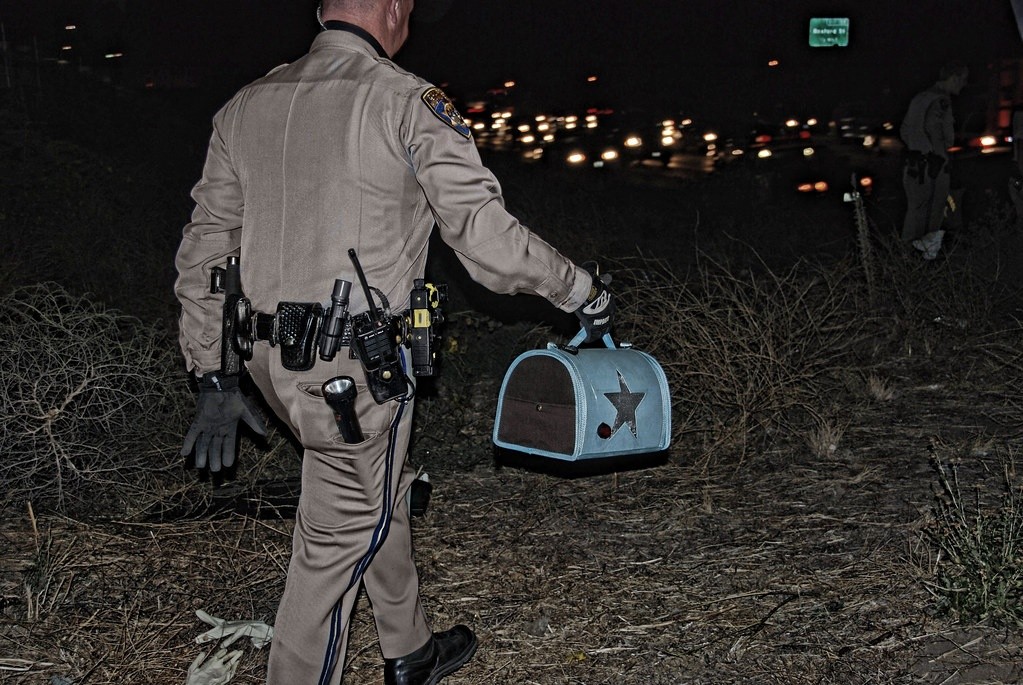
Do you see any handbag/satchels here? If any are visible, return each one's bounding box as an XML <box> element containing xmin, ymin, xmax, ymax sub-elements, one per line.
<box><xmin>493</xmin><ymin>326</ymin><xmax>672</xmax><ymax>462</ymax></box>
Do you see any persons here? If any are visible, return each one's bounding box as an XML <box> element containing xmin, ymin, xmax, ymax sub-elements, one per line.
<box><xmin>1007</xmin><ymin>108</ymin><xmax>1023</xmax><ymax>217</ymax></box>
<box><xmin>174</xmin><ymin>0</ymin><xmax>610</xmax><ymax>685</ymax></box>
<box><xmin>898</xmin><ymin>63</ymin><xmax>969</xmax><ymax>242</ymax></box>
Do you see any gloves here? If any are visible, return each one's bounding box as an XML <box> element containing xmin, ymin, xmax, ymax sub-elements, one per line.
<box><xmin>186</xmin><ymin>649</ymin><xmax>243</xmax><ymax>685</ymax></box>
<box><xmin>575</xmin><ymin>275</ymin><xmax>616</xmax><ymax>342</ymax></box>
<box><xmin>179</xmin><ymin>370</ymin><xmax>269</xmax><ymax>470</ymax></box>
<box><xmin>193</xmin><ymin>610</ymin><xmax>274</xmax><ymax>649</ymax></box>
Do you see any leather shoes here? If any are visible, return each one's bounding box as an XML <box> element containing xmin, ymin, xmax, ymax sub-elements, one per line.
<box><xmin>384</xmin><ymin>624</ymin><xmax>478</xmax><ymax>685</ymax></box>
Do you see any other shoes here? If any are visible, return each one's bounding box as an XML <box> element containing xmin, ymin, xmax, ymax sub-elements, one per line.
<box><xmin>936</xmin><ymin>249</ymin><xmax>945</xmax><ymax>260</ymax></box>
<box><xmin>909</xmin><ymin>239</ymin><xmax>923</xmax><ymax>258</ymax></box>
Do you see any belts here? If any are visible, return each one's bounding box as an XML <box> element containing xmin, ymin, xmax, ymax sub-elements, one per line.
<box><xmin>246</xmin><ymin>309</ymin><xmax>415</xmax><ymax>340</ymax></box>
